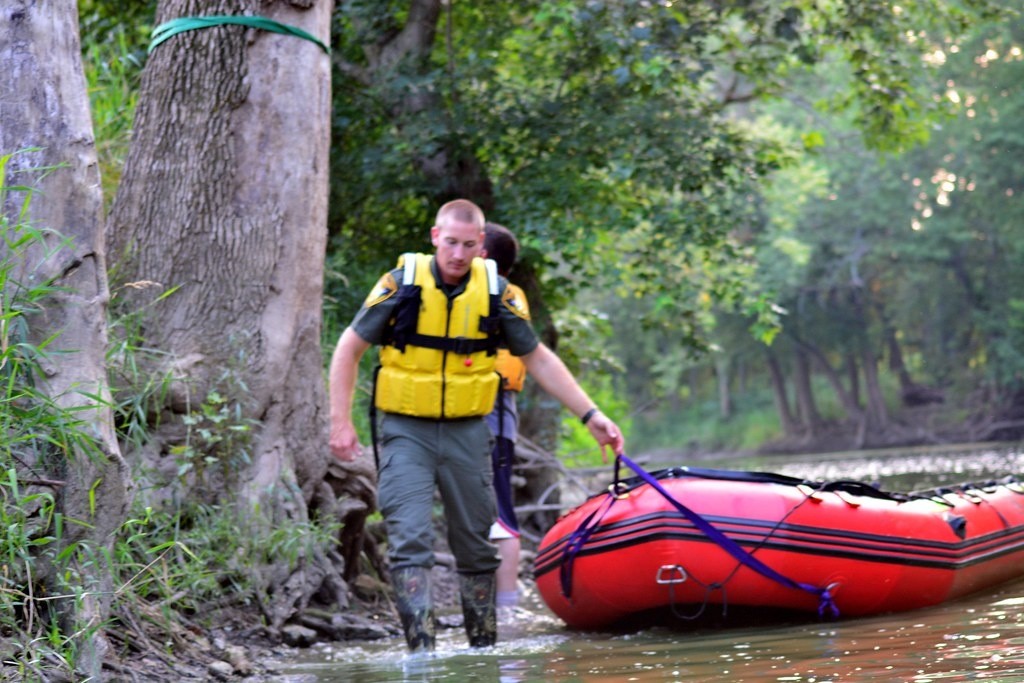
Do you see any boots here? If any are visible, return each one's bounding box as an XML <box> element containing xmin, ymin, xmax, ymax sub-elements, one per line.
<box><xmin>392</xmin><ymin>565</ymin><xmax>434</xmax><ymax>655</ymax></box>
<box><xmin>459</xmin><ymin>573</ymin><xmax>498</xmax><ymax>647</ymax></box>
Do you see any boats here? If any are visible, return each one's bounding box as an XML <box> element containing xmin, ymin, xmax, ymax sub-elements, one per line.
<box><xmin>534</xmin><ymin>465</ymin><xmax>1024</xmax><ymax>632</ymax></box>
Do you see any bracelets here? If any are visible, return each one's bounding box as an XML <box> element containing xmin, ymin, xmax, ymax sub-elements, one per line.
<box><xmin>582</xmin><ymin>408</ymin><xmax>595</xmax><ymax>424</ymax></box>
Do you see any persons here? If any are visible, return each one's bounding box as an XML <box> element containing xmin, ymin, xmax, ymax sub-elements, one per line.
<box><xmin>326</xmin><ymin>199</ymin><xmax>624</xmax><ymax>650</ymax></box>
